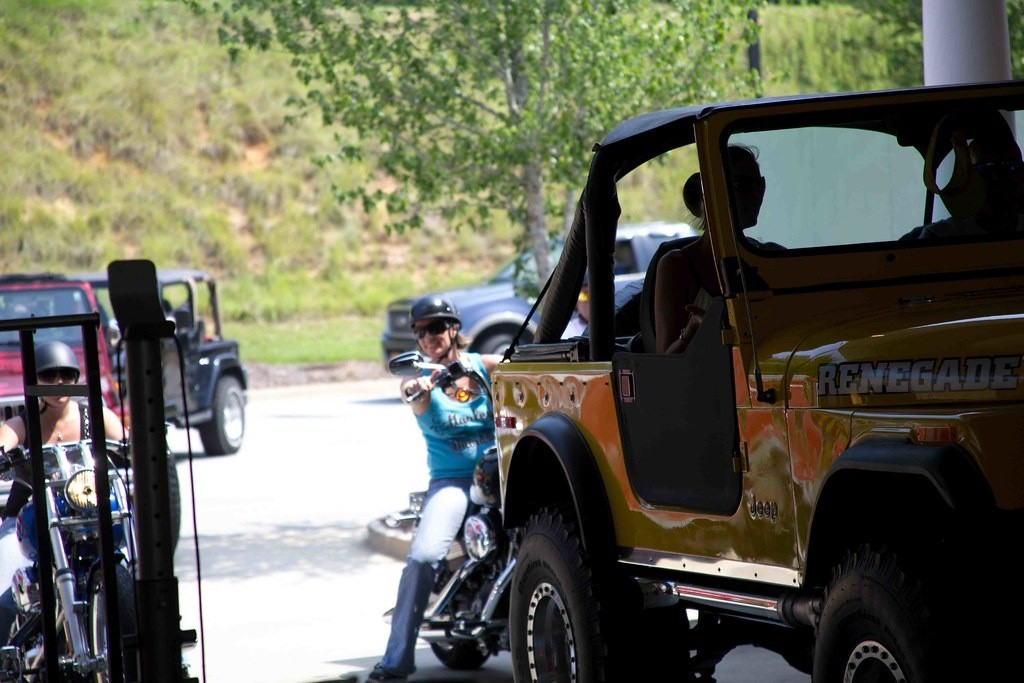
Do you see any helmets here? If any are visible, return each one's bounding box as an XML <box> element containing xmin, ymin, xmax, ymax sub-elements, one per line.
<box><xmin>410</xmin><ymin>292</ymin><xmax>462</xmax><ymax>331</ymax></box>
<box><xmin>34</xmin><ymin>341</ymin><xmax>80</xmax><ymax>377</ymax></box>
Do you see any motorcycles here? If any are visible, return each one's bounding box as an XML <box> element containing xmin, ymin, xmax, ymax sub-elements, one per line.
<box><xmin>0</xmin><ymin>436</ymin><xmax>139</xmax><ymax>682</ymax></box>
<box><xmin>352</xmin><ymin>352</ymin><xmax>519</xmax><ymax>672</ymax></box>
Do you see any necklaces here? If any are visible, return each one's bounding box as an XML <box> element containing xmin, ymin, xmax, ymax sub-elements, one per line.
<box><xmin>56</xmin><ymin>431</ymin><xmax>63</xmax><ymax>441</ymax></box>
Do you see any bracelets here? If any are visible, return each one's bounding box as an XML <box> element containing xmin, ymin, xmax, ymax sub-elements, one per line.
<box><xmin>405</xmin><ymin>386</ymin><xmax>423</xmax><ymax>400</ymax></box>
<box><xmin>680</xmin><ymin>328</ymin><xmax>691</xmax><ymax>343</ymax></box>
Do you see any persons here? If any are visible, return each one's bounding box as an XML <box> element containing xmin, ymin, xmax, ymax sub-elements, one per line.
<box><xmin>0</xmin><ymin>342</ymin><xmax>128</xmax><ymax>673</ymax></box>
<box><xmin>367</xmin><ymin>296</ymin><xmax>511</xmax><ymax>682</ymax></box>
<box><xmin>655</xmin><ymin>143</ymin><xmax>782</xmax><ymax>356</ymax></box>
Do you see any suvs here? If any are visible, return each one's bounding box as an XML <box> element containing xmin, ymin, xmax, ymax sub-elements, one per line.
<box><xmin>486</xmin><ymin>75</ymin><xmax>1024</xmax><ymax>683</ymax></box>
<box><xmin>13</xmin><ymin>267</ymin><xmax>251</xmax><ymax>457</ymax></box>
<box><xmin>0</xmin><ymin>283</ymin><xmax>131</xmax><ymax>447</ymax></box>
<box><xmin>376</xmin><ymin>221</ymin><xmax>701</xmax><ymax>384</ymax></box>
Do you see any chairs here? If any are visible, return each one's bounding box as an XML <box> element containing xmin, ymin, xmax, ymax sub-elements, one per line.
<box><xmin>636</xmin><ymin>235</ymin><xmax>762</xmax><ymax>353</ymax></box>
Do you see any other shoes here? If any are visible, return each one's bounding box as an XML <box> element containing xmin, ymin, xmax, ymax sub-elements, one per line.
<box><xmin>366</xmin><ymin>663</ymin><xmax>408</xmax><ymax>683</ymax></box>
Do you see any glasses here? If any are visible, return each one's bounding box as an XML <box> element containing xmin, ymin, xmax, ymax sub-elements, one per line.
<box><xmin>727</xmin><ymin>176</ymin><xmax>766</xmax><ymax>192</ymax></box>
<box><xmin>37</xmin><ymin>367</ymin><xmax>79</xmax><ymax>384</ymax></box>
<box><xmin>970</xmin><ymin>158</ymin><xmax>1021</xmax><ymax>174</ymax></box>
<box><xmin>413</xmin><ymin>318</ymin><xmax>452</xmax><ymax>340</ymax></box>
